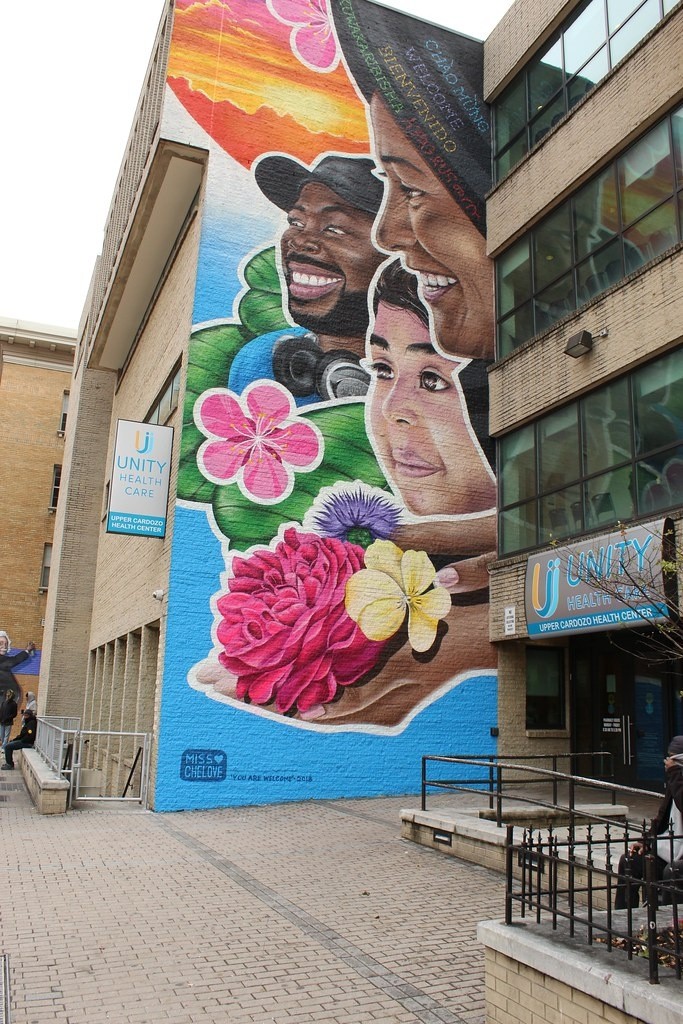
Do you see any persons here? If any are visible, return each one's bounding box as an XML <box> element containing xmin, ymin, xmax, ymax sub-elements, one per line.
<box><xmin>1</xmin><ymin>692</ymin><xmax>37</xmax><ymax>770</ymax></box>
<box><xmin>614</xmin><ymin>735</ymin><xmax>683</xmax><ymax>911</ymax></box>
<box><xmin>0</xmin><ymin>689</ymin><xmax>18</xmax><ymax>753</ymax></box>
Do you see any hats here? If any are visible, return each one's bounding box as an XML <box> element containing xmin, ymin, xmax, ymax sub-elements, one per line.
<box><xmin>668</xmin><ymin>734</ymin><xmax>683</xmax><ymax>756</ymax></box>
<box><xmin>22</xmin><ymin>710</ymin><xmax>35</xmax><ymax>717</ymax></box>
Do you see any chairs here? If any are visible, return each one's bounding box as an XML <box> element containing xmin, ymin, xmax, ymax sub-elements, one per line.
<box><xmin>641</xmin><ymin>458</ymin><xmax>683</xmax><ymax>514</ymax></box>
<box><xmin>522</xmin><ymin>72</ymin><xmax>603</xmax><ymax>156</ymax></box>
<box><xmin>507</xmin><ymin>243</ymin><xmax>652</xmax><ymax>348</ymax></box>
<box><xmin>548</xmin><ymin>492</ymin><xmax>627</xmax><ymax>540</ymax></box>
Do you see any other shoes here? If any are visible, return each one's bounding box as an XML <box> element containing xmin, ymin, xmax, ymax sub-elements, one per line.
<box><xmin>2</xmin><ymin>762</ymin><xmax>14</xmax><ymax>770</ymax></box>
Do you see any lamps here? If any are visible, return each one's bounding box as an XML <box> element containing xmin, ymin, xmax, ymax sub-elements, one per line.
<box><xmin>562</xmin><ymin>330</ymin><xmax>592</xmax><ymax>357</ymax></box>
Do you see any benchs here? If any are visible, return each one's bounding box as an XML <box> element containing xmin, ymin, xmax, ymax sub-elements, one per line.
<box><xmin>16</xmin><ymin>748</ymin><xmax>70</xmax><ymax>814</ymax></box>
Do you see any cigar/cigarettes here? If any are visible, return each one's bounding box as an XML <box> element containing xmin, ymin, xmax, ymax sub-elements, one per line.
<box><xmin>628</xmin><ymin>848</ymin><xmax>636</xmax><ymax>854</ymax></box>
